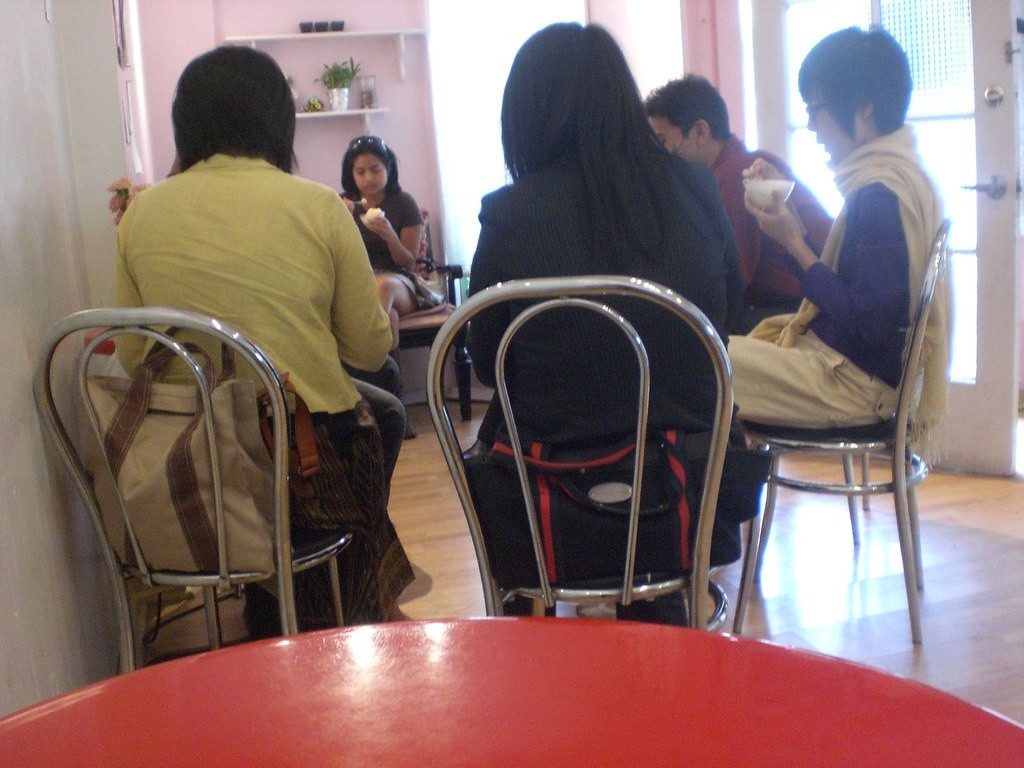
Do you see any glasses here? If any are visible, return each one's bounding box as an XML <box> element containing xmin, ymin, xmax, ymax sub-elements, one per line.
<box><xmin>806</xmin><ymin>102</ymin><xmax>826</xmax><ymax>122</ymax></box>
<box><xmin>349</xmin><ymin>136</ymin><xmax>384</xmax><ymax>152</ymax></box>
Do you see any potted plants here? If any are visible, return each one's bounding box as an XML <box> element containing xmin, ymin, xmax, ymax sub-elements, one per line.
<box><xmin>314</xmin><ymin>55</ymin><xmax>362</xmax><ymax>111</ymax></box>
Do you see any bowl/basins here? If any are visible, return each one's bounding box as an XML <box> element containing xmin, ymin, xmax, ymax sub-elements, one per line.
<box><xmin>742</xmin><ymin>178</ymin><xmax>794</xmax><ymax>212</ymax></box>
<box><xmin>360</xmin><ymin>212</ymin><xmax>385</xmax><ymax>232</ymax></box>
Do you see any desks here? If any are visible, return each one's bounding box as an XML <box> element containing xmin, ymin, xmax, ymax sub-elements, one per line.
<box><xmin>0</xmin><ymin>616</ymin><xmax>1024</xmax><ymax>768</ymax></box>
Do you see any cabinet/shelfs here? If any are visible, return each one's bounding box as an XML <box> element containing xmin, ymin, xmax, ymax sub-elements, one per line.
<box><xmin>224</xmin><ymin>28</ymin><xmax>428</xmax><ymax>136</ymax></box>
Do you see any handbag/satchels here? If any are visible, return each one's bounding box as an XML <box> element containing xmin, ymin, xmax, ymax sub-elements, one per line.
<box><xmin>401</xmin><ymin>257</ymin><xmax>445</xmax><ymax>310</ymax></box>
<box><xmin>75</xmin><ymin>323</ymin><xmax>288</xmax><ymax>576</ymax></box>
<box><xmin>463</xmin><ymin>428</ymin><xmax>770</xmax><ymax>589</ymax></box>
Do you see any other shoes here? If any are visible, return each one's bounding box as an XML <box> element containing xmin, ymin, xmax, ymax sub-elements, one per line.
<box><xmin>405</xmin><ymin>420</ymin><xmax>417</xmax><ymax>439</ymax></box>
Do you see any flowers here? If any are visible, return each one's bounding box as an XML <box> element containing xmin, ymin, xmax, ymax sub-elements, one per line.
<box><xmin>107</xmin><ymin>176</ymin><xmax>151</xmax><ymax>225</ymax></box>
<box><xmin>303</xmin><ymin>94</ymin><xmax>326</xmax><ymax>113</ymax></box>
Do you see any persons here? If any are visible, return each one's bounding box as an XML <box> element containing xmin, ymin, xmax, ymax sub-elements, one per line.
<box><xmin>112</xmin><ymin>46</ymin><xmax>404</xmax><ymax>633</ymax></box>
<box><xmin>339</xmin><ymin>134</ymin><xmax>442</xmax><ymax>438</ymax></box>
<box><xmin>464</xmin><ymin>19</ymin><xmax>951</xmax><ymax>632</ymax></box>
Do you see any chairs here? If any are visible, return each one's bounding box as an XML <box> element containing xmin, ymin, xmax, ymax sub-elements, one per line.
<box><xmin>396</xmin><ymin>262</ymin><xmax>473</xmax><ymax>423</ymax></box>
<box><xmin>732</xmin><ymin>217</ymin><xmax>953</xmax><ymax>645</ymax></box>
<box><xmin>33</xmin><ymin>306</ymin><xmax>354</xmax><ymax>674</ymax></box>
<box><xmin>427</xmin><ymin>276</ymin><xmax>735</xmax><ymax>632</ymax></box>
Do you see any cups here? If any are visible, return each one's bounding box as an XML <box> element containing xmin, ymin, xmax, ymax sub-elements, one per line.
<box><xmin>299</xmin><ymin>22</ymin><xmax>313</xmax><ymax>32</ymax></box>
<box><xmin>315</xmin><ymin>21</ymin><xmax>329</xmax><ymax>32</ymax></box>
<box><xmin>356</xmin><ymin>74</ymin><xmax>378</xmax><ymax>109</ymax></box>
<box><xmin>330</xmin><ymin>21</ymin><xmax>344</xmax><ymax>32</ymax></box>
